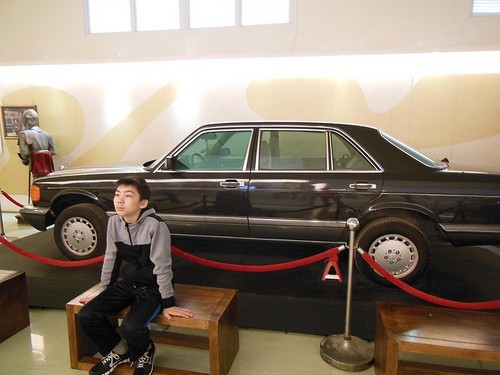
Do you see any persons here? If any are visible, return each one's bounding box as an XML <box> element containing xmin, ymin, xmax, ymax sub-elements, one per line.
<box><xmin>17</xmin><ymin>109</ymin><xmax>58</xmax><ymax>181</ymax></box>
<box><xmin>78</xmin><ymin>177</ymin><xmax>194</xmax><ymax>375</ymax></box>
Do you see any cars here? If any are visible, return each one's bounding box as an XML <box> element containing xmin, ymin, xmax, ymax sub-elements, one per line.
<box><xmin>18</xmin><ymin>120</ymin><xmax>500</xmax><ymax>290</ymax></box>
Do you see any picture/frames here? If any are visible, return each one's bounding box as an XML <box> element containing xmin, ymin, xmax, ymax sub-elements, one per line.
<box><xmin>0</xmin><ymin>105</ymin><xmax>38</xmax><ymax>140</ymax></box>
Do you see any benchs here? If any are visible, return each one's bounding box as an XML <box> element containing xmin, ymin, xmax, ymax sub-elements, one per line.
<box><xmin>66</xmin><ymin>282</ymin><xmax>240</xmax><ymax>375</ymax></box>
<box><xmin>374</xmin><ymin>300</ymin><xmax>500</xmax><ymax>375</ymax></box>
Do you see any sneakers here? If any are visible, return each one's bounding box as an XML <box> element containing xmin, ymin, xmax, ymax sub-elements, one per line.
<box><xmin>133</xmin><ymin>341</ymin><xmax>156</xmax><ymax>375</ymax></box>
<box><xmin>89</xmin><ymin>351</ymin><xmax>130</xmax><ymax>375</ymax></box>
